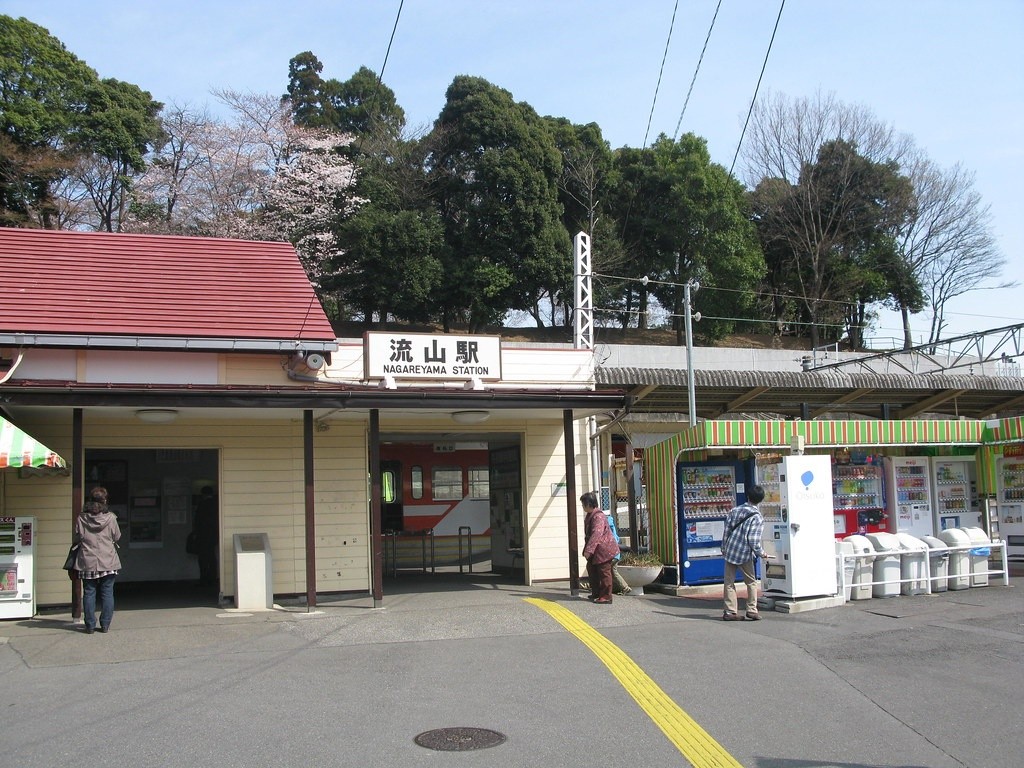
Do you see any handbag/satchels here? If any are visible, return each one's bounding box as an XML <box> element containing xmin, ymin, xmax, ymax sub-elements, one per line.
<box><xmin>62</xmin><ymin>542</ymin><xmax>81</xmax><ymax>570</ymax></box>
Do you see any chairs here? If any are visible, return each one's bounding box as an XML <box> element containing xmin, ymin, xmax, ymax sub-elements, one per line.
<box><xmin>506</xmin><ymin>548</ymin><xmax>525</xmax><ymax>573</ymax></box>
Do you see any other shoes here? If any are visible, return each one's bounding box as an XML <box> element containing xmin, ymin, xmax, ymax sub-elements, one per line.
<box><xmin>595</xmin><ymin>595</ymin><xmax>613</xmax><ymax>604</ymax></box>
<box><xmin>100</xmin><ymin>626</ymin><xmax>108</xmax><ymax>633</ymax></box>
<box><xmin>723</xmin><ymin>612</ymin><xmax>744</xmax><ymax>621</ymax></box>
<box><xmin>746</xmin><ymin>612</ymin><xmax>762</xmax><ymax>620</ymax></box>
<box><xmin>85</xmin><ymin>626</ymin><xmax>94</xmax><ymax>634</ymax></box>
<box><xmin>588</xmin><ymin>594</ymin><xmax>597</xmax><ymax>598</ymax></box>
<box><xmin>617</xmin><ymin>586</ymin><xmax>632</xmax><ymax>595</ymax></box>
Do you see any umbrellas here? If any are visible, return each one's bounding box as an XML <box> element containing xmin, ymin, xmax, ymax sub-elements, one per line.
<box><xmin>0</xmin><ymin>416</ymin><xmax>66</xmax><ymax>469</ymax></box>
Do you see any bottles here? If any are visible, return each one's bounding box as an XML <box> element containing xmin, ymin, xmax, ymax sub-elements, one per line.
<box><xmin>999</xmin><ymin>460</ymin><xmax>1024</xmax><ymax>500</ymax></box>
<box><xmin>937</xmin><ymin>467</ymin><xmax>968</xmax><ymax>509</ymax></box>
<box><xmin>681</xmin><ymin>469</ymin><xmax>736</xmax><ymax>515</ymax></box>
<box><xmin>896</xmin><ymin>467</ymin><xmax>928</xmax><ymax>504</ymax></box>
<box><xmin>755</xmin><ymin>464</ymin><xmax>782</xmax><ymax>522</ymax></box>
<box><xmin>833</xmin><ymin>465</ymin><xmax>883</xmax><ymax>508</ymax></box>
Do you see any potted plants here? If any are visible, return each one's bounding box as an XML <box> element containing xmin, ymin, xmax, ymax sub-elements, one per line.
<box><xmin>612</xmin><ymin>552</ymin><xmax>664</xmax><ymax>596</ymax></box>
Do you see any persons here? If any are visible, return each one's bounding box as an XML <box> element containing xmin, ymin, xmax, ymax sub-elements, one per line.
<box><xmin>74</xmin><ymin>487</ymin><xmax>122</xmax><ymax>634</ymax></box>
<box><xmin>194</xmin><ymin>486</ymin><xmax>219</xmax><ymax>586</ymax></box>
<box><xmin>720</xmin><ymin>486</ymin><xmax>769</xmax><ymax>621</ymax></box>
<box><xmin>581</xmin><ymin>493</ymin><xmax>632</xmax><ymax>605</ymax></box>
